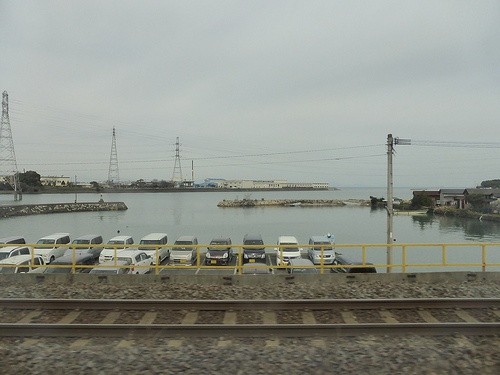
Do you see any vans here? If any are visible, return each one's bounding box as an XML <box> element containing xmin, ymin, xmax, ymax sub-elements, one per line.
<box><xmin>99</xmin><ymin>236</ymin><xmax>133</xmax><ymax>264</ymax></box>
<box><xmin>0</xmin><ymin>236</ymin><xmax>26</xmax><ymax>244</ymax></box>
<box><xmin>31</xmin><ymin>232</ymin><xmax>71</xmax><ymax>263</ymax></box>
<box><xmin>242</xmin><ymin>232</ymin><xmax>266</xmax><ymax>264</ymax></box>
<box><xmin>0</xmin><ymin>254</ymin><xmax>48</xmax><ymax>275</ymax></box>
<box><xmin>45</xmin><ymin>254</ymin><xmax>95</xmax><ymax>274</ymax></box>
<box><xmin>309</xmin><ymin>236</ymin><xmax>336</xmax><ymax>264</ymax></box>
<box><xmin>169</xmin><ymin>235</ymin><xmax>199</xmax><ymax>266</ymax></box>
<box><xmin>286</xmin><ymin>258</ymin><xmax>318</xmax><ymax>273</ymax></box>
<box><xmin>63</xmin><ymin>235</ymin><xmax>103</xmax><ymax>261</ymax></box>
<box><xmin>0</xmin><ymin>245</ymin><xmax>30</xmax><ymax>261</ymax></box>
<box><xmin>89</xmin><ymin>260</ymin><xmax>133</xmax><ymax>275</ymax></box>
<box><xmin>205</xmin><ymin>237</ymin><xmax>233</xmax><ymax>266</ymax></box>
<box><xmin>274</xmin><ymin>235</ymin><xmax>303</xmax><ymax>266</ymax></box>
<box><xmin>138</xmin><ymin>232</ymin><xmax>169</xmax><ymax>265</ymax></box>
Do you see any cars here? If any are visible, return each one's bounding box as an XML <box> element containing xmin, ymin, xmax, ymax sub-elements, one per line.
<box><xmin>112</xmin><ymin>249</ymin><xmax>153</xmax><ymax>275</ymax></box>
<box><xmin>346</xmin><ymin>262</ymin><xmax>377</xmax><ymax>274</ymax></box>
<box><xmin>242</xmin><ymin>262</ymin><xmax>271</xmax><ymax>274</ymax></box>
